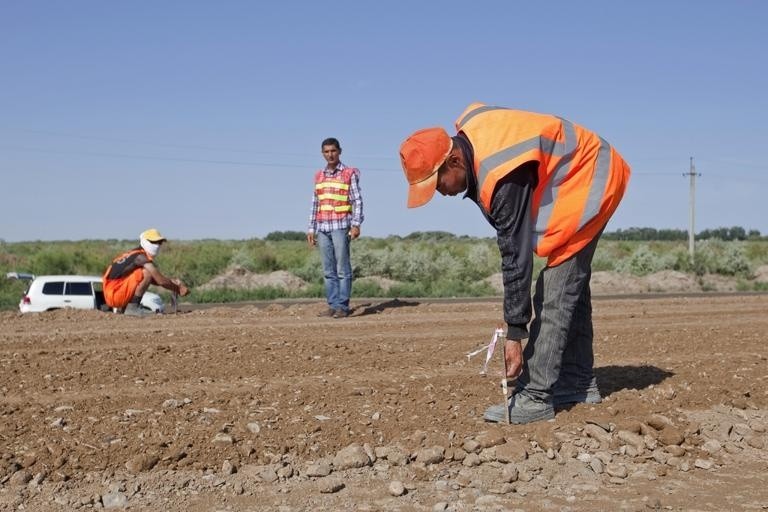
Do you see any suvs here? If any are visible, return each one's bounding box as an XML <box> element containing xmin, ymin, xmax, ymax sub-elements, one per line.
<box><xmin>3</xmin><ymin>274</ymin><xmax>169</xmax><ymax>320</ymax></box>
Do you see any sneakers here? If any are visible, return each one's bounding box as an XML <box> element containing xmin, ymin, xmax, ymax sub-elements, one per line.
<box><xmin>333</xmin><ymin>308</ymin><xmax>348</xmax><ymax>318</ymax></box>
<box><xmin>316</xmin><ymin>306</ymin><xmax>335</xmax><ymax>318</ymax></box>
<box><xmin>123</xmin><ymin>302</ymin><xmax>157</xmax><ymax>317</ymax></box>
<box><xmin>550</xmin><ymin>375</ymin><xmax>603</xmax><ymax>405</ymax></box>
<box><xmin>484</xmin><ymin>392</ymin><xmax>556</xmax><ymax>426</ymax></box>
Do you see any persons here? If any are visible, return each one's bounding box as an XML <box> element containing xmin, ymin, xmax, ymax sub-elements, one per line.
<box><xmin>102</xmin><ymin>229</ymin><xmax>191</xmax><ymax>317</ymax></box>
<box><xmin>399</xmin><ymin>102</ymin><xmax>632</xmax><ymax>427</ymax></box>
<box><xmin>306</xmin><ymin>138</ymin><xmax>364</xmax><ymax>319</ymax></box>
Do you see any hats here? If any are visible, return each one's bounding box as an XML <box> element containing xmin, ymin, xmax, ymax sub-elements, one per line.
<box><xmin>143</xmin><ymin>227</ymin><xmax>168</xmax><ymax>244</ymax></box>
<box><xmin>399</xmin><ymin>126</ymin><xmax>454</xmax><ymax>209</ymax></box>
